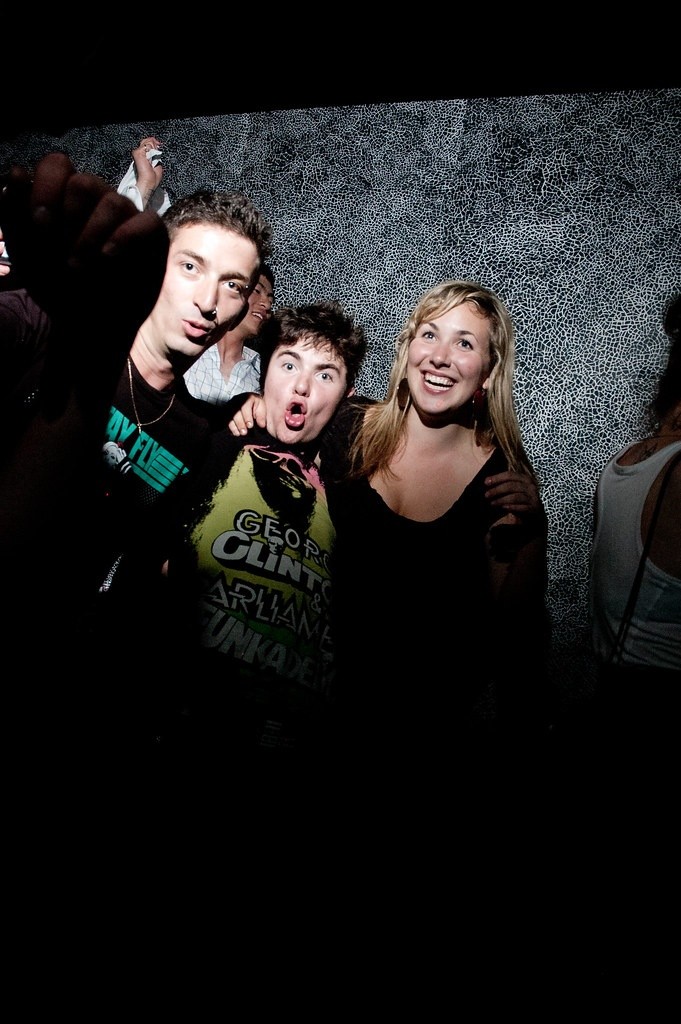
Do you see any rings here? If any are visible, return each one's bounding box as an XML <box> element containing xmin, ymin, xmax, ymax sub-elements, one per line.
<box><xmin>145</xmin><ymin>145</ymin><xmax>147</xmax><ymax>151</ymax></box>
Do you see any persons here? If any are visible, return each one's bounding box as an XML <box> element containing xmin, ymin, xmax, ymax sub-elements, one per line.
<box><xmin>0</xmin><ymin>136</ymin><xmax>550</xmax><ymax>727</ymax></box>
<box><xmin>587</xmin><ymin>295</ymin><xmax>681</xmax><ymax>720</ymax></box>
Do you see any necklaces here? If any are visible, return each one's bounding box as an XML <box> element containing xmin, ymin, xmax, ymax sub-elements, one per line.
<box><xmin>127</xmin><ymin>359</ymin><xmax>175</xmax><ymax>437</ymax></box>
